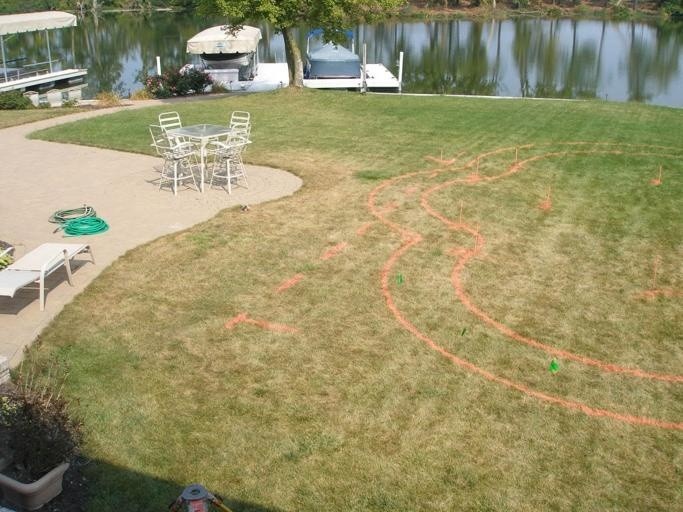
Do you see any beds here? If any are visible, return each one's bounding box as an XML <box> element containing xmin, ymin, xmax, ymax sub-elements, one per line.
<box><xmin>0</xmin><ymin>242</ymin><xmax>95</xmax><ymax>312</ymax></box>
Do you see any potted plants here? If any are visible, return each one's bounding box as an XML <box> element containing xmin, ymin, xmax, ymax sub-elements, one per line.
<box><xmin>0</xmin><ymin>343</ymin><xmax>85</xmax><ymax>511</ymax></box>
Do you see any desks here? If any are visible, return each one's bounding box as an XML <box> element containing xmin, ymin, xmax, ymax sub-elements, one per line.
<box><xmin>166</xmin><ymin>124</ymin><xmax>242</xmax><ymax>184</ymax></box>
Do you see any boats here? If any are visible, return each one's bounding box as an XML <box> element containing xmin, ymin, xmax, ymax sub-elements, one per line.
<box><xmin>0</xmin><ymin>10</ymin><xmax>89</xmax><ymax>93</ymax></box>
<box><xmin>302</xmin><ymin>27</ymin><xmax>361</xmax><ymax>79</ymax></box>
<box><xmin>185</xmin><ymin>24</ymin><xmax>263</xmax><ymax>80</ymax></box>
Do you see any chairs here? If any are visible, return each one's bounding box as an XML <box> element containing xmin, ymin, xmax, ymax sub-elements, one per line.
<box><xmin>206</xmin><ymin>128</ymin><xmax>248</xmax><ymax>195</ymax></box>
<box><xmin>212</xmin><ymin>111</ymin><xmax>250</xmax><ymax>173</ymax></box>
<box><xmin>159</xmin><ymin>111</ymin><xmax>201</xmax><ymax>178</ymax></box>
<box><xmin>148</xmin><ymin>125</ymin><xmax>200</xmax><ymax>196</ymax></box>
